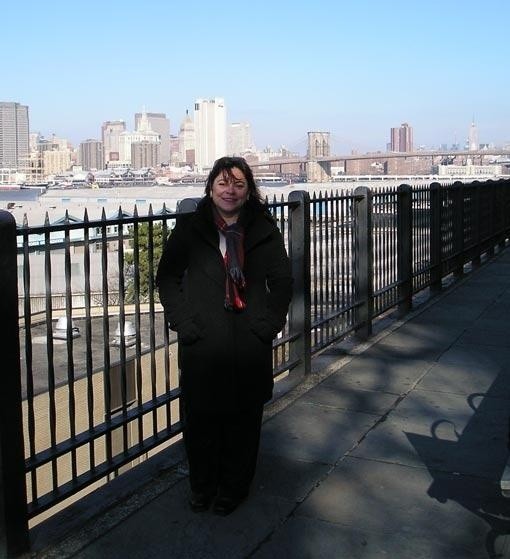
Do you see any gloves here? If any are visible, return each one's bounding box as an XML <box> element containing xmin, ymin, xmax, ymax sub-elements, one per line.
<box><xmin>173</xmin><ymin>319</ymin><xmax>206</xmax><ymax>346</ymax></box>
<box><xmin>249</xmin><ymin>320</ymin><xmax>279</xmax><ymax>344</ymax></box>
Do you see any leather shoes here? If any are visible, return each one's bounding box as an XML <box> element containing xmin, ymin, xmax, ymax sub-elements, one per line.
<box><xmin>213</xmin><ymin>495</ymin><xmax>244</xmax><ymax>516</ymax></box>
<box><xmin>188</xmin><ymin>490</ymin><xmax>212</xmax><ymax>513</ymax></box>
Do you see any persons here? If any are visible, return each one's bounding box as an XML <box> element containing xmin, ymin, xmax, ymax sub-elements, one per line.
<box><xmin>158</xmin><ymin>156</ymin><xmax>293</xmax><ymax>517</ymax></box>
